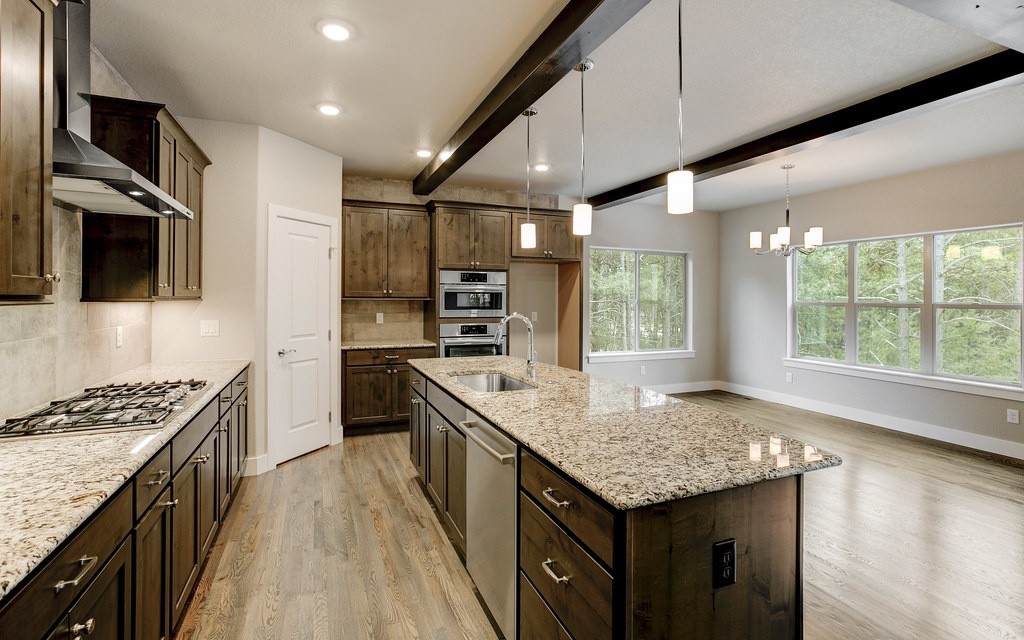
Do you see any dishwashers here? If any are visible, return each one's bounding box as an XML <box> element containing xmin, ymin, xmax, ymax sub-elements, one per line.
<box><xmin>461</xmin><ymin>409</ymin><xmax>520</xmax><ymax>640</ymax></box>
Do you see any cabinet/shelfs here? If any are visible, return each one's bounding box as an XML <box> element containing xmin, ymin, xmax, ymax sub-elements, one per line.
<box><xmin>430</xmin><ymin>206</ymin><xmax>512</xmax><ymax>269</ymax></box>
<box><xmin>0</xmin><ymin>0</ymin><xmax>61</xmax><ymax>303</ymax></box>
<box><xmin>340</xmin><ymin>198</ymin><xmax>431</xmax><ymax>301</ymax></box>
<box><xmin>511</xmin><ymin>211</ymin><xmax>582</xmax><ymax>263</ymax></box>
<box><xmin>340</xmin><ymin>347</ymin><xmax>435</xmax><ymax>435</ymax></box>
<box><xmin>515</xmin><ymin>445</ymin><xmax>618</xmax><ymax>639</ymax></box>
<box><xmin>407</xmin><ymin>366</ymin><xmax>469</xmax><ymax>560</ymax></box>
<box><xmin>77</xmin><ymin>100</ymin><xmax>212</xmax><ymax>302</ymax></box>
<box><xmin>0</xmin><ymin>366</ymin><xmax>251</xmax><ymax>640</ymax></box>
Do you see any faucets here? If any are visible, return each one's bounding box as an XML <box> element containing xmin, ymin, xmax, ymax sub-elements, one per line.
<box><xmin>493</xmin><ymin>311</ymin><xmax>537</xmax><ymax>367</ymax></box>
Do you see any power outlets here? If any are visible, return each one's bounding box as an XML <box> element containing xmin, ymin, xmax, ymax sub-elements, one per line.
<box><xmin>712</xmin><ymin>538</ymin><xmax>737</xmax><ymax>591</ymax></box>
<box><xmin>1006</xmin><ymin>409</ymin><xmax>1020</xmax><ymax>424</ymax></box>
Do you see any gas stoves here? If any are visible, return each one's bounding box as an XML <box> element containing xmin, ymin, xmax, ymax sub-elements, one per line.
<box><xmin>0</xmin><ymin>377</ymin><xmax>215</xmax><ymax>442</ymax></box>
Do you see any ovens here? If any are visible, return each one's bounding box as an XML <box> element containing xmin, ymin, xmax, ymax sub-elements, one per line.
<box><xmin>439</xmin><ymin>270</ymin><xmax>507</xmax><ymax>318</ymax></box>
<box><xmin>439</xmin><ymin>323</ymin><xmax>508</xmax><ymax>358</ymax></box>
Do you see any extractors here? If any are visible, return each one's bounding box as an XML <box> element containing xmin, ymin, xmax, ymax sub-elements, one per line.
<box><xmin>51</xmin><ymin>1</ymin><xmax>195</xmax><ymax>221</ymax></box>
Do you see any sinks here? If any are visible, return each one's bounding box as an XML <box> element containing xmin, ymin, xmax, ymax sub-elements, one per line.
<box><xmin>449</xmin><ymin>373</ymin><xmax>538</xmax><ymax>393</ymax></box>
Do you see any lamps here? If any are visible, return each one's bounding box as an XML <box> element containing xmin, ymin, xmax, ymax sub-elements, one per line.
<box><xmin>520</xmin><ymin>108</ymin><xmax>537</xmax><ymax>250</ymax></box>
<box><xmin>666</xmin><ymin>1</ymin><xmax>694</xmax><ymax>215</ymax></box>
<box><xmin>572</xmin><ymin>58</ymin><xmax>593</xmax><ymax>236</ymax></box>
<box><xmin>750</xmin><ymin>163</ymin><xmax>824</xmax><ymax>256</ymax></box>
